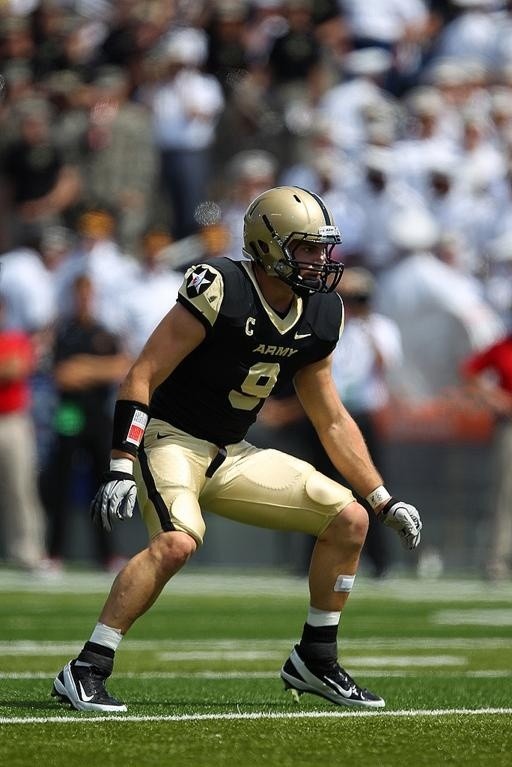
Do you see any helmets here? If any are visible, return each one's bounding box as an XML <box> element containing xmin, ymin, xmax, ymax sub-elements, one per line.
<box><xmin>242</xmin><ymin>185</ymin><xmax>342</xmax><ymax>278</ymax></box>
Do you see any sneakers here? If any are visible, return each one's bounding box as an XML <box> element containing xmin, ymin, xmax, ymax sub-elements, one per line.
<box><xmin>51</xmin><ymin>658</ymin><xmax>127</xmax><ymax>712</ymax></box>
<box><xmin>280</xmin><ymin>644</ymin><xmax>385</xmax><ymax>708</ymax></box>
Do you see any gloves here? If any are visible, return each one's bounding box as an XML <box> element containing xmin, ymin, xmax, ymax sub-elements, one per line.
<box><xmin>377</xmin><ymin>499</ymin><xmax>422</xmax><ymax>552</ymax></box>
<box><xmin>88</xmin><ymin>470</ymin><xmax>137</xmax><ymax>532</ymax></box>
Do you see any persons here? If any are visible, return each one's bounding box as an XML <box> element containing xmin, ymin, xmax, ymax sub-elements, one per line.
<box><xmin>1</xmin><ymin>1</ymin><xmax>512</xmax><ymax>573</ymax></box>
<box><xmin>52</xmin><ymin>184</ymin><xmax>422</xmax><ymax>706</ymax></box>
